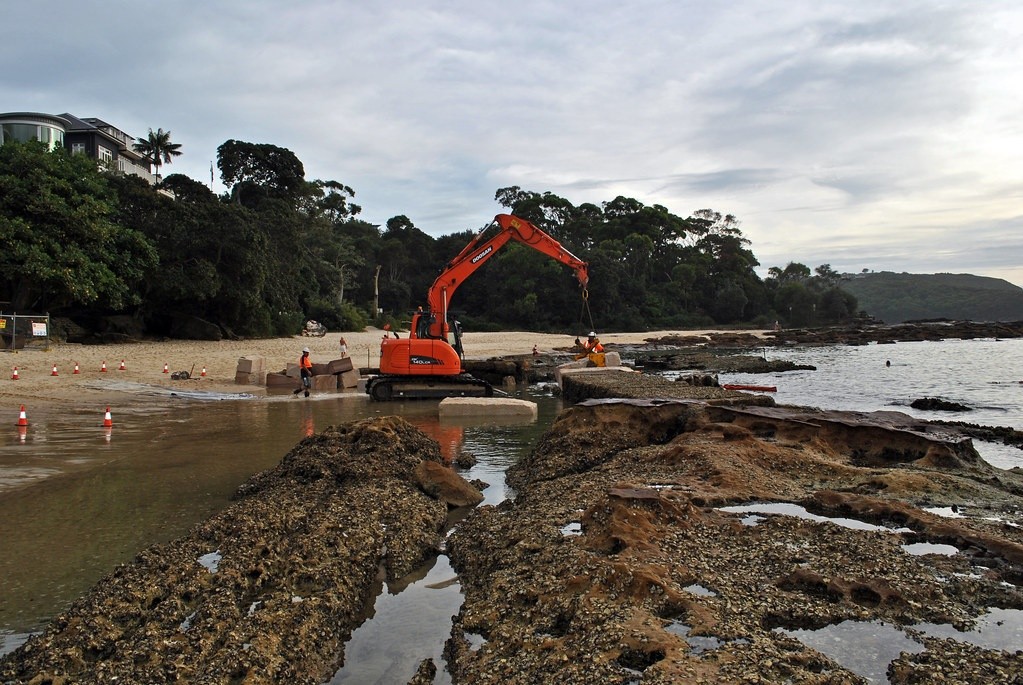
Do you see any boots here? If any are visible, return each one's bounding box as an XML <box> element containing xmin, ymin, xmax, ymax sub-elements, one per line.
<box><xmin>293</xmin><ymin>387</ymin><xmax>302</xmax><ymax>398</ymax></box>
<box><xmin>305</xmin><ymin>392</ymin><xmax>309</xmax><ymax>397</ymax></box>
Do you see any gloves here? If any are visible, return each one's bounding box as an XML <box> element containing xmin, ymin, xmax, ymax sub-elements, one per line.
<box><xmin>575</xmin><ymin>339</ymin><xmax>580</xmax><ymax>345</ymax></box>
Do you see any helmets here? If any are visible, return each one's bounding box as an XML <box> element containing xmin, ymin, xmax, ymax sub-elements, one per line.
<box><xmin>588</xmin><ymin>332</ymin><xmax>596</xmax><ymax>336</ymax></box>
<box><xmin>302</xmin><ymin>348</ymin><xmax>309</xmax><ymax>352</ymax></box>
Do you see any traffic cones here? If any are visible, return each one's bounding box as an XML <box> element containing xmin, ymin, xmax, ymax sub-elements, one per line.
<box><xmin>118</xmin><ymin>359</ymin><xmax>127</xmax><ymax>370</ymax></box>
<box><xmin>100</xmin><ymin>361</ymin><xmax>108</xmax><ymax>372</ymax></box>
<box><xmin>103</xmin><ymin>426</ymin><xmax>113</xmax><ymax>445</ymax></box>
<box><xmin>14</xmin><ymin>405</ymin><xmax>30</xmax><ymax>426</ymax></box>
<box><xmin>50</xmin><ymin>363</ymin><xmax>59</xmax><ymax>376</ymax></box>
<box><xmin>162</xmin><ymin>363</ymin><xmax>169</xmax><ymax>373</ymax></box>
<box><xmin>11</xmin><ymin>367</ymin><xmax>20</xmax><ymax>380</ymax></box>
<box><xmin>16</xmin><ymin>425</ymin><xmax>29</xmax><ymax>445</ymax></box>
<box><xmin>100</xmin><ymin>407</ymin><xmax>112</xmax><ymax>427</ymax></box>
<box><xmin>72</xmin><ymin>362</ymin><xmax>81</xmax><ymax>374</ymax></box>
<box><xmin>200</xmin><ymin>366</ymin><xmax>207</xmax><ymax>376</ymax></box>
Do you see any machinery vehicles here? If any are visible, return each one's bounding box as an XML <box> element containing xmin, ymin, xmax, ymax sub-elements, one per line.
<box><xmin>364</xmin><ymin>213</ymin><xmax>604</xmax><ymax>403</ymax></box>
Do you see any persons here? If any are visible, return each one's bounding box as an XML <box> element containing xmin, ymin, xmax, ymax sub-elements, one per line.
<box><xmin>575</xmin><ymin>331</ymin><xmax>606</xmax><ymax>367</ymax></box>
<box><xmin>293</xmin><ymin>347</ymin><xmax>313</xmax><ymax>398</ymax></box>
<box><xmin>340</xmin><ymin>337</ymin><xmax>347</xmax><ymax>359</ymax></box>
<box><xmin>532</xmin><ymin>344</ymin><xmax>538</xmax><ymax>356</ymax></box>
